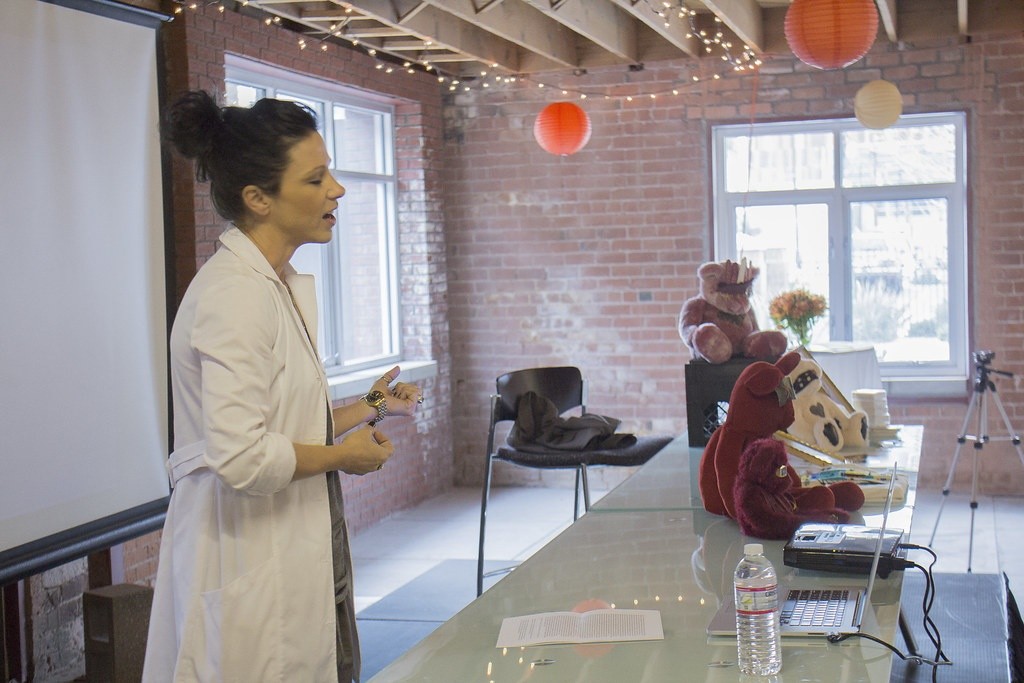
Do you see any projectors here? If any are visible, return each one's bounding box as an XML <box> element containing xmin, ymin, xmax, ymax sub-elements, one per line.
<box><xmin>783</xmin><ymin>522</ymin><xmax>905</xmax><ymax>578</ymax></box>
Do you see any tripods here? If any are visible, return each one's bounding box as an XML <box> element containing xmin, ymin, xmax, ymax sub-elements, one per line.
<box><xmin>930</xmin><ymin>364</ymin><xmax>1024</xmax><ymax>574</ymax></box>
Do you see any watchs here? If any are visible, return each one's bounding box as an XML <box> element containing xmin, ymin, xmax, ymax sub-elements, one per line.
<box><xmin>358</xmin><ymin>390</ymin><xmax>387</xmax><ymax>423</ymax></box>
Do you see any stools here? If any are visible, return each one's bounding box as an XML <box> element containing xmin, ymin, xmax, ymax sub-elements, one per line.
<box><xmin>476</xmin><ymin>367</ymin><xmax>674</xmax><ymax>597</ymax></box>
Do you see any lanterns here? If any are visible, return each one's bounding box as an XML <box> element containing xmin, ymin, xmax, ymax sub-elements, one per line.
<box><xmin>851</xmin><ymin>78</ymin><xmax>903</xmax><ymax>130</ymax></box>
<box><xmin>532</xmin><ymin>101</ymin><xmax>593</xmax><ymax>156</ymax></box>
<box><xmin>784</xmin><ymin>0</ymin><xmax>879</xmax><ymax>71</ymax></box>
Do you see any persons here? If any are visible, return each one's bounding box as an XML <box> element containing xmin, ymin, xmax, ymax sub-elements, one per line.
<box><xmin>142</xmin><ymin>90</ymin><xmax>422</xmax><ymax>683</ymax></box>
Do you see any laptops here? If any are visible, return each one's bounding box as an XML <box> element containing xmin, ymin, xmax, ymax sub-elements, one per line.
<box><xmin>706</xmin><ymin>462</ymin><xmax>897</xmax><ymax>637</ymax></box>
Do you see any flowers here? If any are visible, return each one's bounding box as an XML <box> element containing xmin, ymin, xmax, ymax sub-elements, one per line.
<box><xmin>769</xmin><ymin>287</ymin><xmax>828</xmax><ymax>346</ymax></box>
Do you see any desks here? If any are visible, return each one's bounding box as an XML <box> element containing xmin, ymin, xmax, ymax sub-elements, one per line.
<box><xmin>360</xmin><ymin>506</ymin><xmax>913</xmax><ymax>683</ymax></box>
<box><xmin>811</xmin><ymin>344</ymin><xmax>886</xmax><ymax>410</ymax></box>
<box><xmin>554</xmin><ymin>424</ymin><xmax>924</xmax><ymax>657</ymax></box>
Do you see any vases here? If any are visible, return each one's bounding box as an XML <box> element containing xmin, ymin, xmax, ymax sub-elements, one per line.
<box><xmin>788</xmin><ymin>327</ymin><xmax>813</xmax><ymax>347</ymax></box>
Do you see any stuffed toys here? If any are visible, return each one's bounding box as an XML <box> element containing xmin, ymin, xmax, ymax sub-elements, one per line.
<box><xmin>678</xmin><ymin>260</ymin><xmax>789</xmax><ymax>365</ymax></box>
<box><xmin>697</xmin><ymin>352</ymin><xmax>869</xmax><ymax>540</ymax></box>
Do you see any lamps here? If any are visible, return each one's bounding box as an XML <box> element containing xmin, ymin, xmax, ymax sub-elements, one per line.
<box><xmin>534</xmin><ymin>101</ymin><xmax>592</xmax><ymax>158</ymax></box>
<box><xmin>785</xmin><ymin>0</ymin><xmax>879</xmax><ymax>71</ymax></box>
<box><xmin>852</xmin><ymin>81</ymin><xmax>903</xmax><ymax>132</ymax></box>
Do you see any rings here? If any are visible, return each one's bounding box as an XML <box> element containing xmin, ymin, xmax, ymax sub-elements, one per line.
<box><xmin>381</xmin><ymin>374</ymin><xmax>393</xmax><ymax>384</ymax></box>
<box><xmin>377</xmin><ymin>463</ymin><xmax>384</xmax><ymax>470</ymax></box>
<box><xmin>417</xmin><ymin>395</ymin><xmax>425</xmax><ymax>404</ymax></box>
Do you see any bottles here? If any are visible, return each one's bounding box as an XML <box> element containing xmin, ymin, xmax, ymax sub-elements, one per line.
<box><xmin>733</xmin><ymin>543</ymin><xmax>783</xmax><ymax>675</ymax></box>
